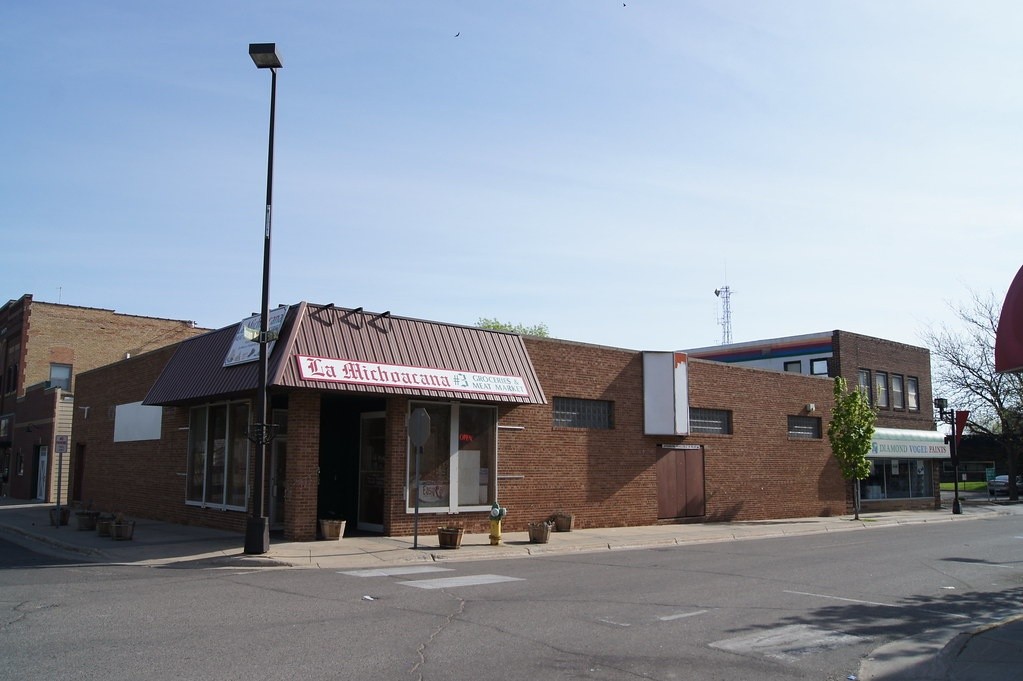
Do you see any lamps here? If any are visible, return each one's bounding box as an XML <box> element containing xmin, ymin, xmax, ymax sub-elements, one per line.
<box><xmin>24</xmin><ymin>422</ymin><xmax>40</xmax><ymax>432</ymax></box>
<box><xmin>807</xmin><ymin>404</ymin><xmax>816</xmax><ymax>411</ymax></box>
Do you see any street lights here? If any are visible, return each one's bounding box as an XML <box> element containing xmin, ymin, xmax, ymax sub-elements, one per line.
<box><xmin>244</xmin><ymin>42</ymin><xmax>282</xmax><ymax>554</ymax></box>
<box><xmin>935</xmin><ymin>398</ymin><xmax>963</xmax><ymax>514</ymax></box>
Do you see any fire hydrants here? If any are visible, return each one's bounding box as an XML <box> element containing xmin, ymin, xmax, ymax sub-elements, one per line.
<box><xmin>488</xmin><ymin>502</ymin><xmax>506</xmax><ymax>545</ymax></box>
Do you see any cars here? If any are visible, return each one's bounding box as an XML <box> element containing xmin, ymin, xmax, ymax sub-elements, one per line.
<box><xmin>988</xmin><ymin>475</ymin><xmax>1023</xmax><ymax>496</ymax></box>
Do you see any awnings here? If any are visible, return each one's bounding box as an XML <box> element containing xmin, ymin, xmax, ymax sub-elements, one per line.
<box><xmin>994</xmin><ymin>264</ymin><xmax>1023</xmax><ymax>375</ymax></box>
<box><xmin>863</xmin><ymin>428</ymin><xmax>951</xmax><ymax>459</ymax></box>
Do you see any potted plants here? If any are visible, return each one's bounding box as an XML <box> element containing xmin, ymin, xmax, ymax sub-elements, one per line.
<box><xmin>437</xmin><ymin>524</ymin><xmax>464</xmax><ymax>549</ymax></box>
<box><xmin>527</xmin><ymin>521</ymin><xmax>555</xmax><ymax>544</ymax></box>
<box><xmin>75</xmin><ymin>496</ymin><xmax>100</xmax><ymax>531</ymax></box>
<box><xmin>96</xmin><ymin>515</ymin><xmax>111</xmax><ymax>537</ymax></box>
<box><xmin>551</xmin><ymin>513</ymin><xmax>575</xmax><ymax>532</ymax></box>
<box><xmin>109</xmin><ymin>512</ymin><xmax>135</xmax><ymax>540</ymax></box>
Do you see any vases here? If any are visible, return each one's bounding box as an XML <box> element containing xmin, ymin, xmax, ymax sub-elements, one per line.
<box><xmin>319</xmin><ymin>519</ymin><xmax>346</xmax><ymax>540</ymax></box>
<box><xmin>49</xmin><ymin>507</ymin><xmax>70</xmax><ymax>526</ymax></box>
